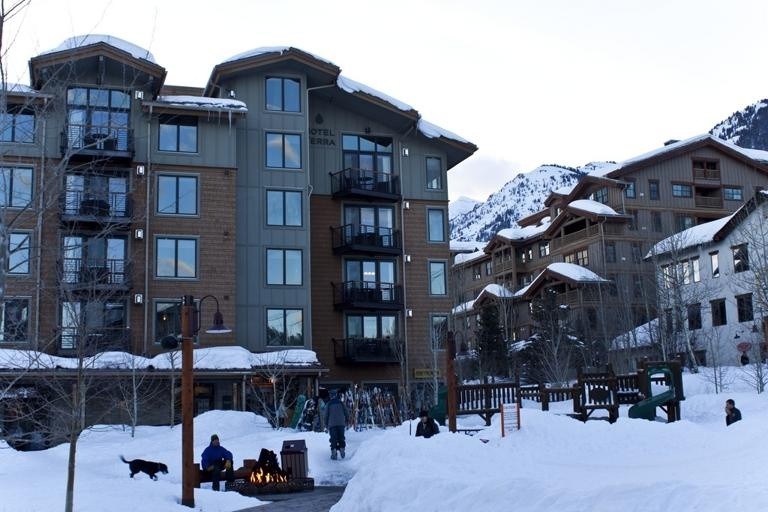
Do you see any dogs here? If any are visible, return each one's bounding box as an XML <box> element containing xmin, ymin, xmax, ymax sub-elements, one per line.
<box><xmin>119</xmin><ymin>455</ymin><xmax>168</xmax><ymax>481</ymax></box>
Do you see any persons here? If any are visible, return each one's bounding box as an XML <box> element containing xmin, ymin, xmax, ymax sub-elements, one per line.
<box><xmin>415</xmin><ymin>410</ymin><xmax>439</xmax><ymax>438</ymax></box>
<box><xmin>740</xmin><ymin>352</ymin><xmax>749</xmax><ymax>366</ymax></box>
<box><xmin>323</xmin><ymin>390</ymin><xmax>349</xmax><ymax>460</ymax></box>
<box><xmin>278</xmin><ymin>399</ymin><xmax>288</xmax><ymax>427</ymax></box>
<box><xmin>201</xmin><ymin>434</ymin><xmax>235</xmax><ymax>491</ymax></box>
<box><xmin>725</xmin><ymin>399</ymin><xmax>742</xmax><ymax>426</ymax></box>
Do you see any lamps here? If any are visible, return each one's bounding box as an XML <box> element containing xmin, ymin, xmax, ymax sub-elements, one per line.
<box><xmin>734</xmin><ymin>330</ymin><xmax>744</xmax><ymax>339</ymax></box>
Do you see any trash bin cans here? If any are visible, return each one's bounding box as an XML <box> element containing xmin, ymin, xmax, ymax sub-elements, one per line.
<box><xmin>280</xmin><ymin>439</ymin><xmax>308</xmax><ymax>478</ymax></box>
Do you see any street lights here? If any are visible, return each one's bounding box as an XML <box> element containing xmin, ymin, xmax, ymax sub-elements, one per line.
<box><xmin>445</xmin><ymin>328</ymin><xmax>471</xmax><ymax>434</ymax></box>
<box><xmin>160</xmin><ymin>292</ymin><xmax>231</xmax><ymax>510</ymax></box>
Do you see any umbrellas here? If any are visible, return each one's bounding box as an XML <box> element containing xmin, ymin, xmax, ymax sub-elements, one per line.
<box><xmin>737</xmin><ymin>343</ymin><xmax>752</xmax><ymax>351</ymax></box>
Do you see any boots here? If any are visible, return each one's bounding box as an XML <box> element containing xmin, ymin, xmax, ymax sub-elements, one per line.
<box><xmin>339</xmin><ymin>448</ymin><xmax>345</xmax><ymax>458</ymax></box>
<box><xmin>330</xmin><ymin>448</ymin><xmax>336</xmax><ymax>459</ymax></box>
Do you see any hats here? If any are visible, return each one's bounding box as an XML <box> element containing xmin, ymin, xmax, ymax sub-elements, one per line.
<box><xmin>211</xmin><ymin>435</ymin><xmax>218</xmax><ymax>441</ymax></box>
<box><xmin>418</xmin><ymin>411</ymin><xmax>427</xmax><ymax>418</ymax></box>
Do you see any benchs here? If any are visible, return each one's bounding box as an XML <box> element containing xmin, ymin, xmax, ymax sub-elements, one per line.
<box><xmin>193</xmin><ymin>460</ymin><xmax>256</xmax><ymax>488</ymax></box>
